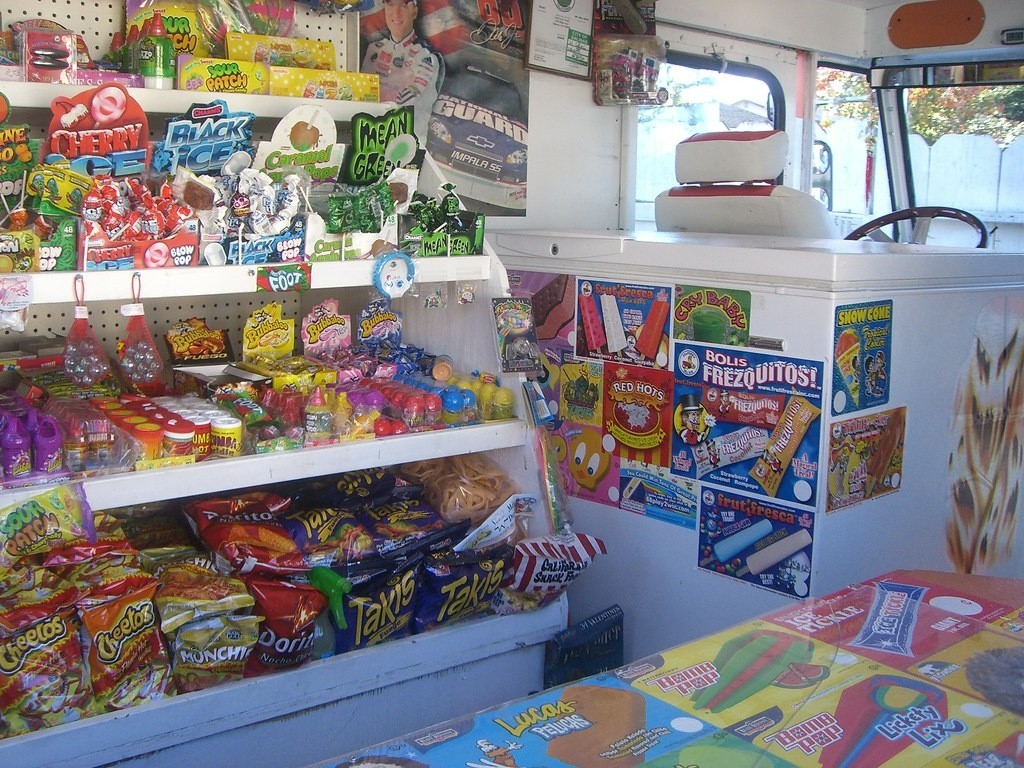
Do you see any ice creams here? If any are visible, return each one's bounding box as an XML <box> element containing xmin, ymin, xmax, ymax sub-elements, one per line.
<box><xmin>735</xmin><ymin>527</ymin><xmax>812</xmax><ymax>578</ymax></box>
<box><xmin>698</xmin><ymin>519</ymin><xmax>774</xmax><ymax>566</ymax></box>
<box><xmin>578</xmin><ymin>292</ymin><xmax>670</xmax><ymax>366</ymax></box>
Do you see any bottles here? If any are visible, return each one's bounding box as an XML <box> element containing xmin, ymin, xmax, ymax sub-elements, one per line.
<box><xmin>304</xmin><ymin>386</ymin><xmax>332</xmax><ymax>438</ymax></box>
<box><xmin>333</xmin><ymin>391</ymin><xmax>353</xmax><ymax>428</ymax></box>
<box><xmin>102</xmin><ymin>13</ymin><xmax>174</xmax><ymax>90</ymax></box>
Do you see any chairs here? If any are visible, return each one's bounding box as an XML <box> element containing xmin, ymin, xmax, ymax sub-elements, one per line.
<box><xmin>654</xmin><ymin>128</ymin><xmax>843</xmax><ymax>242</ymax></box>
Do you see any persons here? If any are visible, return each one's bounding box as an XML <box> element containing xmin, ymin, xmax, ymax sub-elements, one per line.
<box><xmin>359</xmin><ymin>0</ymin><xmax>445</xmax><ymax>150</ymax></box>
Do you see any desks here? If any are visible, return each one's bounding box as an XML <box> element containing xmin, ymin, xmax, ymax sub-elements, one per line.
<box><xmin>303</xmin><ymin>573</ymin><xmax>1024</xmax><ymax>768</ymax></box>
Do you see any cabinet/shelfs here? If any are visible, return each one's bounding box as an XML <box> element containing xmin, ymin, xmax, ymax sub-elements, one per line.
<box><xmin>0</xmin><ymin>80</ymin><xmax>569</xmax><ymax>768</ymax></box>
